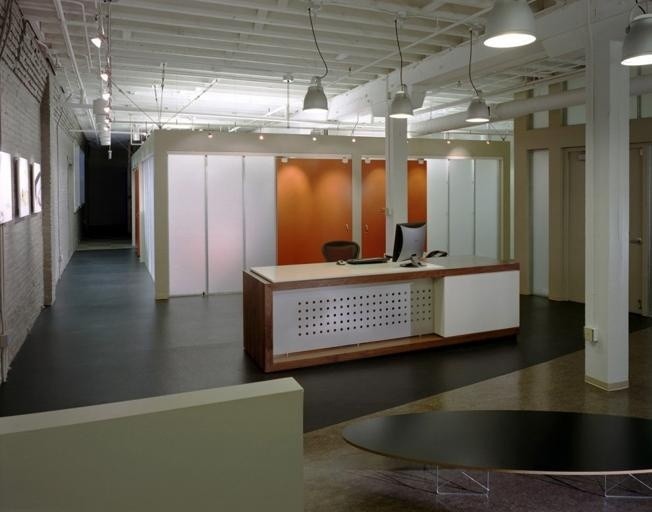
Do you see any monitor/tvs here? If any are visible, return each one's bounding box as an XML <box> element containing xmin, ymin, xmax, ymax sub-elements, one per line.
<box><xmin>392</xmin><ymin>221</ymin><xmax>427</xmax><ymax>268</ymax></box>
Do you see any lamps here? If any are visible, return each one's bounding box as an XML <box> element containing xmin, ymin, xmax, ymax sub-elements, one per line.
<box><xmin>620</xmin><ymin>1</ymin><xmax>652</xmax><ymax>66</ymax></box>
<box><xmin>302</xmin><ymin>1</ymin><xmax>537</xmax><ymax>123</ymax></box>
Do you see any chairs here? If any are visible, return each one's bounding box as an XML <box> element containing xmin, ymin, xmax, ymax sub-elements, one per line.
<box><xmin>322</xmin><ymin>241</ymin><xmax>360</xmax><ymax>264</ymax></box>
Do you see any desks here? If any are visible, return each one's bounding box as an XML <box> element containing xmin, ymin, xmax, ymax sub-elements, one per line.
<box><xmin>242</xmin><ymin>254</ymin><xmax>521</xmax><ymax>374</ymax></box>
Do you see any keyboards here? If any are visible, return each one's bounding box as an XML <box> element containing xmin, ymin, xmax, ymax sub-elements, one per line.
<box><xmin>347</xmin><ymin>257</ymin><xmax>387</xmax><ymax>264</ymax></box>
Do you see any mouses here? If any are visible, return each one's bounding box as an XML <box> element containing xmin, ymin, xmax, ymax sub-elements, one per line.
<box><xmin>337</xmin><ymin>259</ymin><xmax>346</xmax><ymax>265</ymax></box>
